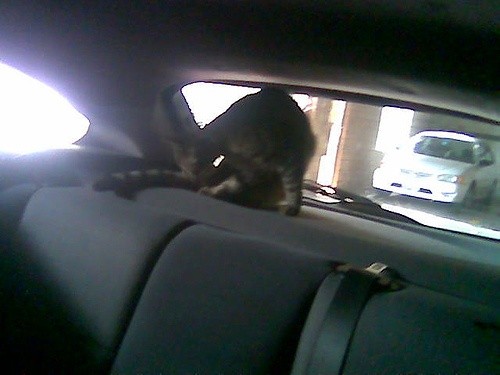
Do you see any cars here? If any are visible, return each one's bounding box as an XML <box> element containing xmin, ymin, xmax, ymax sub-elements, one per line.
<box><xmin>372</xmin><ymin>129</ymin><xmax>499</xmax><ymax>211</ymax></box>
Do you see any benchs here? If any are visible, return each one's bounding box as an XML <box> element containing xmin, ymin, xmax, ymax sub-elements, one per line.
<box><xmin>4</xmin><ymin>178</ymin><xmax>500</xmax><ymax>371</ymax></box>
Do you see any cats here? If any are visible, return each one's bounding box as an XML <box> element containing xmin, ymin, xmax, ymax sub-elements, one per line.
<box><xmin>91</xmin><ymin>87</ymin><xmax>317</xmax><ymax>218</ymax></box>
